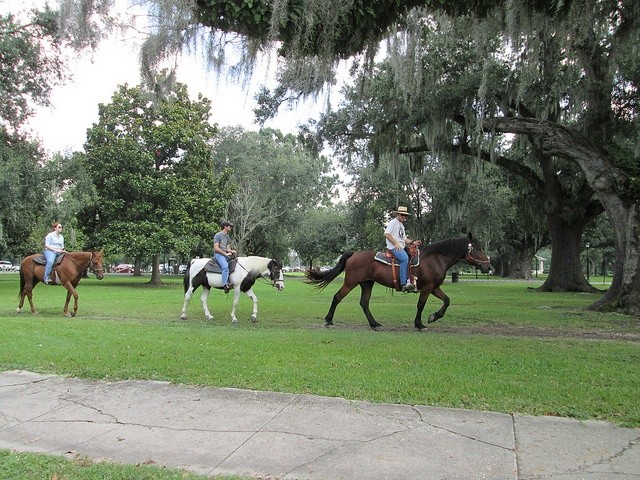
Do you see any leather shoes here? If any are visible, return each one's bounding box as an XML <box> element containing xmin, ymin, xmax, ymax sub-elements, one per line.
<box><xmin>224</xmin><ymin>284</ymin><xmax>232</xmax><ymax>293</ymax></box>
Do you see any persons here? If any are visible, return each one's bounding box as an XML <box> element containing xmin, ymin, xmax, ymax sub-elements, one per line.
<box><xmin>213</xmin><ymin>221</ymin><xmax>236</xmax><ymax>294</ymax></box>
<box><xmin>383</xmin><ymin>206</ymin><xmax>422</xmax><ymax>289</ymax></box>
<box><xmin>43</xmin><ymin>222</ymin><xmax>65</xmax><ymax>285</ymax></box>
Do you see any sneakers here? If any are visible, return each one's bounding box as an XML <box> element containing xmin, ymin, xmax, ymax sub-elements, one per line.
<box><xmin>402</xmin><ymin>283</ymin><xmax>415</xmax><ymax>289</ymax></box>
<box><xmin>44</xmin><ymin>279</ymin><xmax>52</xmax><ymax>283</ymax></box>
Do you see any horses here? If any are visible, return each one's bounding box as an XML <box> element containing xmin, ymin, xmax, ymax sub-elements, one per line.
<box><xmin>16</xmin><ymin>248</ymin><xmax>104</xmax><ymax>318</ymax></box>
<box><xmin>180</xmin><ymin>255</ymin><xmax>285</xmax><ymax>328</ymax></box>
<box><xmin>303</xmin><ymin>227</ymin><xmax>496</xmax><ymax>332</ymax></box>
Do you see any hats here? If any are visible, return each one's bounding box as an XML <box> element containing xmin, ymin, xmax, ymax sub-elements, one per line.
<box><xmin>394</xmin><ymin>206</ymin><xmax>412</xmax><ymax>216</ymax></box>
<box><xmin>221</xmin><ymin>221</ymin><xmax>233</xmax><ymax>226</ymax></box>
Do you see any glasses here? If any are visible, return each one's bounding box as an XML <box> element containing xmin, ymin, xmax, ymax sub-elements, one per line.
<box><xmin>59</xmin><ymin>226</ymin><xmax>63</xmax><ymax>228</ymax></box>
<box><xmin>401</xmin><ymin>214</ymin><xmax>407</xmax><ymax>216</ymax></box>
